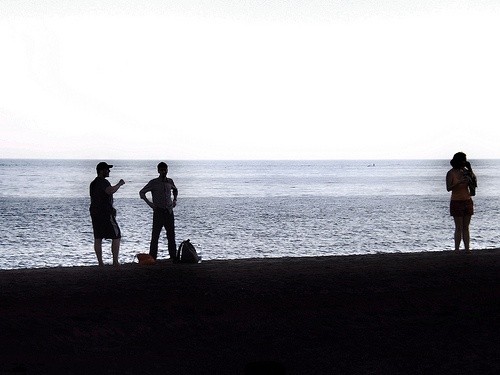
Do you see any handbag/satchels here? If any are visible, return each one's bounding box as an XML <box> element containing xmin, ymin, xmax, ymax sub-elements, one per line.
<box><xmin>136</xmin><ymin>253</ymin><xmax>154</xmax><ymax>264</ymax></box>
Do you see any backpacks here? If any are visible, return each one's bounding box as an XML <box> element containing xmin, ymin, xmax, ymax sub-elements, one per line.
<box><xmin>177</xmin><ymin>240</ymin><xmax>201</xmax><ymax>263</ymax></box>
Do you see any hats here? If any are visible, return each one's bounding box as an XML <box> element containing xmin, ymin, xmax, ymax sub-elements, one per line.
<box><xmin>96</xmin><ymin>162</ymin><xmax>113</xmax><ymax>169</ymax></box>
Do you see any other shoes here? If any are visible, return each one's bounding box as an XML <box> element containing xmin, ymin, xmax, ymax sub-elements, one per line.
<box><xmin>113</xmin><ymin>262</ymin><xmax>122</xmax><ymax>268</ymax></box>
<box><xmin>453</xmin><ymin>250</ymin><xmax>460</xmax><ymax>257</ymax></box>
<box><xmin>99</xmin><ymin>263</ymin><xmax>109</xmax><ymax>268</ymax></box>
<box><xmin>170</xmin><ymin>256</ymin><xmax>179</xmax><ymax>261</ymax></box>
<box><xmin>465</xmin><ymin>250</ymin><xmax>475</xmax><ymax>255</ymax></box>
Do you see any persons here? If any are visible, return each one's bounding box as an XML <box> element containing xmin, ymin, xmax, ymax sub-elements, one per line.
<box><xmin>139</xmin><ymin>161</ymin><xmax>179</xmax><ymax>261</ymax></box>
<box><xmin>445</xmin><ymin>152</ymin><xmax>478</xmax><ymax>251</ymax></box>
<box><xmin>89</xmin><ymin>162</ymin><xmax>125</xmax><ymax>266</ymax></box>
